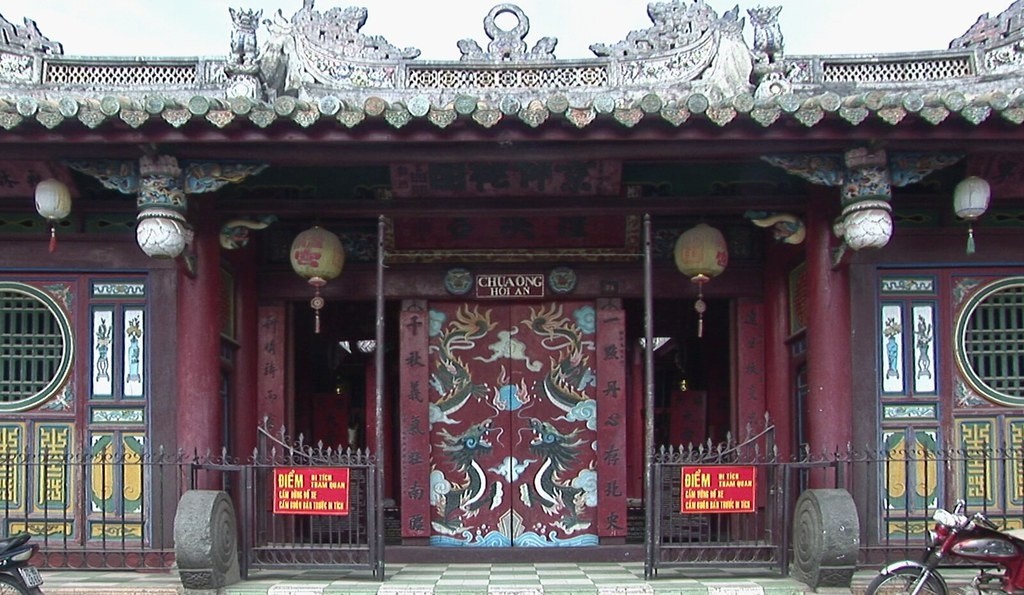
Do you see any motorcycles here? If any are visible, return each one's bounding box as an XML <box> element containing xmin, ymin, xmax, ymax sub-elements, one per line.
<box><xmin>1</xmin><ymin>531</ymin><xmax>44</xmax><ymax>595</ymax></box>
<box><xmin>863</xmin><ymin>499</ymin><xmax>1024</xmax><ymax>595</ymax></box>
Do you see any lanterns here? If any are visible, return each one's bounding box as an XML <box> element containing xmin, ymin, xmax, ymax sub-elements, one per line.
<box><xmin>674</xmin><ymin>223</ymin><xmax>728</xmax><ymax>337</ymax></box>
<box><xmin>290</xmin><ymin>226</ymin><xmax>344</xmax><ymax>332</ymax></box>
<box><xmin>954</xmin><ymin>176</ymin><xmax>990</xmax><ymax>253</ymax></box>
<box><xmin>35</xmin><ymin>175</ymin><xmax>71</xmax><ymax>253</ymax></box>
